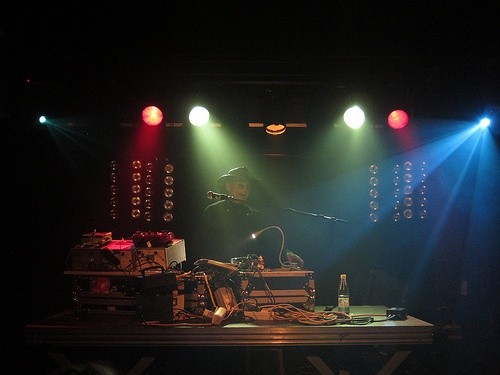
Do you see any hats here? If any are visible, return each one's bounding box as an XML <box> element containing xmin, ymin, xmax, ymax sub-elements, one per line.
<box><xmin>218</xmin><ymin>167</ymin><xmax>263</xmax><ymax>188</ymax></box>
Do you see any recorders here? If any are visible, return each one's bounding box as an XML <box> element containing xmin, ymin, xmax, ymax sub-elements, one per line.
<box><xmin>132</xmin><ymin>231</ymin><xmax>173</xmax><ymax>247</ymax></box>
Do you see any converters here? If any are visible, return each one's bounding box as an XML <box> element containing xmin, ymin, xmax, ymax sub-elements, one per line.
<box><xmin>387</xmin><ymin>307</ymin><xmax>408</xmax><ymax>320</ymax></box>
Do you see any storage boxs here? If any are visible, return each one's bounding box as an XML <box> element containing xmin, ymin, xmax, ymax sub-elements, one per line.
<box><xmin>234</xmin><ymin>269</ymin><xmax>318</xmax><ymax>320</ymax></box>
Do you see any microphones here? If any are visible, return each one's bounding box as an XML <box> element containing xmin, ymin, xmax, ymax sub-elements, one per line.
<box><xmin>207</xmin><ymin>191</ymin><xmax>233</xmax><ymax>201</ymax></box>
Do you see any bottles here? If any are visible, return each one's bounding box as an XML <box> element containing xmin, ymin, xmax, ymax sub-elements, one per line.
<box><xmin>338</xmin><ymin>274</ymin><xmax>350</xmax><ymax>315</ymax></box>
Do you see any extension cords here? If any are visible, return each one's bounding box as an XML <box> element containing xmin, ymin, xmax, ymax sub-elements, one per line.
<box><xmin>212</xmin><ymin>307</ymin><xmax>226</xmax><ymax>324</ymax></box>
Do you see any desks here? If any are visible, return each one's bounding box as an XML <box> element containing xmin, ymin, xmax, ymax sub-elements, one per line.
<box><xmin>22</xmin><ymin>304</ymin><xmax>436</xmax><ymax>375</ymax></box>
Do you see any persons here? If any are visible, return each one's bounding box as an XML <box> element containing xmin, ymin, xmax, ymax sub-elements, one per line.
<box><xmin>196</xmin><ymin>165</ymin><xmax>305</xmax><ymax>274</ymax></box>
